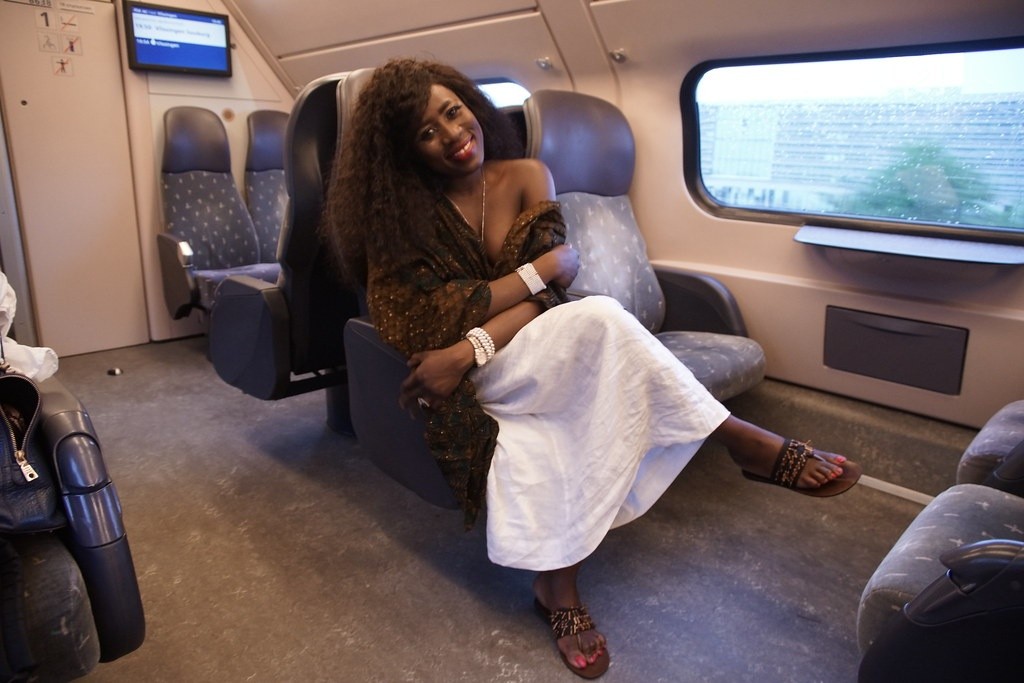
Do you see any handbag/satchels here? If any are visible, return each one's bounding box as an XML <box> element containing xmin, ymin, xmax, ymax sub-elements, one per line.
<box><xmin>0</xmin><ymin>372</ymin><xmax>67</xmax><ymax>533</ymax></box>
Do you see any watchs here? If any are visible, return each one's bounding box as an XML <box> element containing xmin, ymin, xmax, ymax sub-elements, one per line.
<box><xmin>467</xmin><ymin>336</ymin><xmax>487</xmax><ymax>368</ymax></box>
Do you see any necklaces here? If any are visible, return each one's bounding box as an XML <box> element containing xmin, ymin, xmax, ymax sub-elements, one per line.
<box><xmin>433</xmin><ymin>163</ymin><xmax>486</xmax><ymax>247</ymax></box>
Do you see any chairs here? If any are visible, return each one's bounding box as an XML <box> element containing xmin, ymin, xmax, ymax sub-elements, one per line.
<box><xmin>204</xmin><ymin>63</ymin><xmax>360</xmax><ymax>407</ymax></box>
<box><xmin>518</xmin><ymin>89</ymin><xmax>767</xmax><ymax>406</ymax></box>
<box><xmin>147</xmin><ymin>104</ymin><xmax>317</xmax><ymax>322</ymax></box>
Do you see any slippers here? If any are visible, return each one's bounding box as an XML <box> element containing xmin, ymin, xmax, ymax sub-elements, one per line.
<box><xmin>532</xmin><ymin>598</ymin><xmax>610</xmax><ymax>679</ymax></box>
<box><xmin>741</xmin><ymin>437</ymin><xmax>862</xmax><ymax>496</ymax></box>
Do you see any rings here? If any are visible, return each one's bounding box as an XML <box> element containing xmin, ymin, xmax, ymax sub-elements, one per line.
<box><xmin>417</xmin><ymin>397</ymin><xmax>431</xmax><ymax>410</ymax></box>
<box><xmin>566</xmin><ymin>242</ymin><xmax>572</xmax><ymax>248</ymax></box>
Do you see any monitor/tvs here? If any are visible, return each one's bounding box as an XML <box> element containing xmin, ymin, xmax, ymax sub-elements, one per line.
<box><xmin>123</xmin><ymin>0</ymin><xmax>233</xmax><ymax>77</ymax></box>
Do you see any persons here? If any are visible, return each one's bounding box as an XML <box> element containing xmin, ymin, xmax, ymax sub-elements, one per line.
<box><xmin>320</xmin><ymin>59</ymin><xmax>862</xmax><ymax>679</ymax></box>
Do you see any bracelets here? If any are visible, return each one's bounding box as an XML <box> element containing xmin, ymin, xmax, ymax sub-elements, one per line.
<box><xmin>465</xmin><ymin>327</ymin><xmax>496</xmax><ymax>367</ymax></box>
<box><xmin>512</xmin><ymin>264</ymin><xmax>547</xmax><ymax>294</ymax></box>
